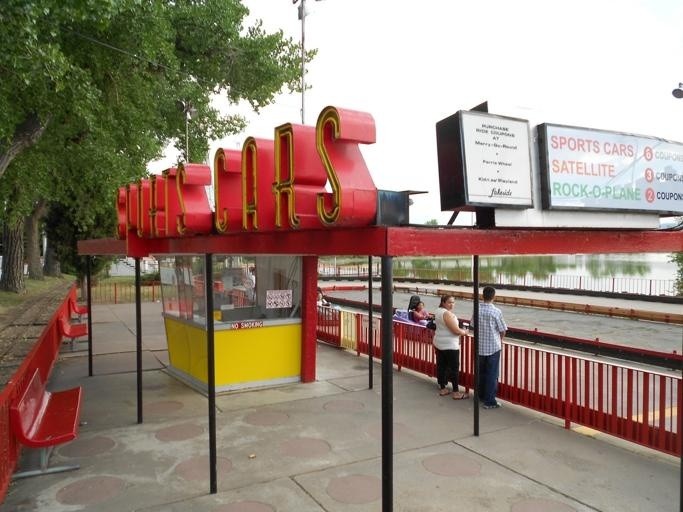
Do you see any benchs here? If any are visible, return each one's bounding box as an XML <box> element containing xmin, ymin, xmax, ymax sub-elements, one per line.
<box><xmin>7</xmin><ymin>366</ymin><xmax>83</xmax><ymax>480</ymax></box>
<box><xmin>57</xmin><ymin>294</ymin><xmax>89</xmax><ymax>355</ymax></box>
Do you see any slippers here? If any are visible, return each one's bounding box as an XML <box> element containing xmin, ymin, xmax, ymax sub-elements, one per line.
<box><xmin>440</xmin><ymin>390</ymin><xmax>468</xmax><ymax>399</ymax></box>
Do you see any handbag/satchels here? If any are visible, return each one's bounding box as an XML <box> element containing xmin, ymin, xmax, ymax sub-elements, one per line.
<box><xmin>427</xmin><ymin>321</ymin><xmax>436</xmax><ymax>329</ymax></box>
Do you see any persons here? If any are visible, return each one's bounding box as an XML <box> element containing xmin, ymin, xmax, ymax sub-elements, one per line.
<box><xmin>433</xmin><ymin>295</ymin><xmax>470</xmax><ymax>399</ymax></box>
<box><xmin>409</xmin><ymin>302</ymin><xmax>435</xmax><ymax>327</ymax></box>
<box><xmin>316</xmin><ymin>291</ymin><xmax>335</xmax><ymax>315</ymax></box>
<box><xmin>469</xmin><ymin>286</ymin><xmax>508</xmax><ymax>409</ymax></box>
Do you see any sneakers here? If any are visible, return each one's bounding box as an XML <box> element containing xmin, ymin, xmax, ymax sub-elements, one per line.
<box><xmin>484</xmin><ymin>402</ymin><xmax>500</xmax><ymax>409</ymax></box>
